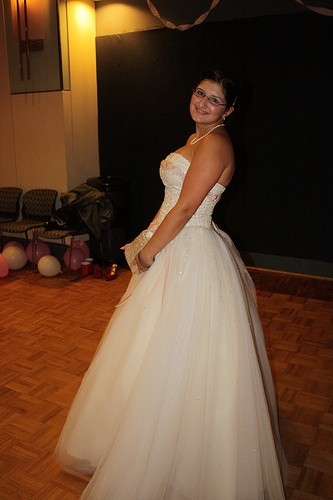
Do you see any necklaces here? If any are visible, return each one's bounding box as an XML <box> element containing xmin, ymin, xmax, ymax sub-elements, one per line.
<box><xmin>189</xmin><ymin>123</ymin><xmax>225</xmax><ymax>145</ymax></box>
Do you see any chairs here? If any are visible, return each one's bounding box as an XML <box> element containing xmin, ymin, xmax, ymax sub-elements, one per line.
<box><xmin>32</xmin><ymin>209</ymin><xmax>78</xmax><ymax>274</ymax></box>
<box><xmin>0</xmin><ymin>187</ymin><xmax>22</xmax><ymax>223</ymax></box>
<box><xmin>0</xmin><ymin>189</ymin><xmax>58</xmax><ymax>244</ymax></box>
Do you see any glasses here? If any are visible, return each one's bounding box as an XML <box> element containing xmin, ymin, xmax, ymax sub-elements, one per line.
<box><xmin>193</xmin><ymin>88</ymin><xmax>226</xmax><ymax>107</ymax></box>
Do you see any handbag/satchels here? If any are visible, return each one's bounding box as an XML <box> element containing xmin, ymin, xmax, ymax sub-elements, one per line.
<box><xmin>52</xmin><ymin>189</ymin><xmax>96</xmax><ymax>228</ymax></box>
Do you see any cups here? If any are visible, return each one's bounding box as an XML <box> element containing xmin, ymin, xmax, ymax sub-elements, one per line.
<box><xmin>84</xmin><ymin>258</ymin><xmax>94</xmax><ymax>273</ymax></box>
<box><xmin>81</xmin><ymin>262</ymin><xmax>90</xmax><ymax>276</ymax></box>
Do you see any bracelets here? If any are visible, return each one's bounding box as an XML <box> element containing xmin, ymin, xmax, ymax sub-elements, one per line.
<box><xmin>136</xmin><ymin>250</ymin><xmax>156</xmax><ymax>268</ymax></box>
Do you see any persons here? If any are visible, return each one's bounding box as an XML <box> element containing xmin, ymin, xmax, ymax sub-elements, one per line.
<box><xmin>52</xmin><ymin>69</ymin><xmax>292</xmax><ymax>500</ymax></box>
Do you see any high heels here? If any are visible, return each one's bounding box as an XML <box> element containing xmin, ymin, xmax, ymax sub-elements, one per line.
<box><xmin>93</xmin><ymin>264</ymin><xmax>102</xmax><ymax>278</ymax></box>
<box><xmin>104</xmin><ymin>263</ymin><xmax>118</xmax><ymax>280</ymax></box>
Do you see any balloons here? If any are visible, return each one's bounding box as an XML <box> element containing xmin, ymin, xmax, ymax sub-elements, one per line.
<box><xmin>0</xmin><ymin>252</ymin><xmax>9</xmax><ymax>278</ymax></box>
<box><xmin>26</xmin><ymin>240</ymin><xmax>52</xmax><ymax>264</ymax></box>
<box><xmin>70</xmin><ymin>242</ymin><xmax>90</xmax><ymax>258</ymax></box>
<box><xmin>2</xmin><ymin>245</ymin><xmax>28</xmax><ymax>271</ymax></box>
<box><xmin>64</xmin><ymin>247</ymin><xmax>85</xmax><ymax>271</ymax></box>
<box><xmin>3</xmin><ymin>241</ymin><xmax>25</xmax><ymax>256</ymax></box>
<box><xmin>37</xmin><ymin>255</ymin><xmax>63</xmax><ymax>277</ymax></box>
